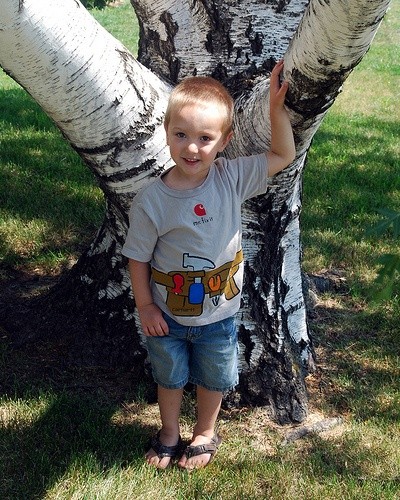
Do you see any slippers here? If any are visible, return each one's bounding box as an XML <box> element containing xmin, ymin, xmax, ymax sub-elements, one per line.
<box><xmin>144</xmin><ymin>433</ymin><xmax>182</xmax><ymax>474</ymax></box>
<box><xmin>178</xmin><ymin>434</ymin><xmax>223</xmax><ymax>471</ymax></box>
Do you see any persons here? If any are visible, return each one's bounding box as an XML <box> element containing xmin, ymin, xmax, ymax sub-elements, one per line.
<box><xmin>120</xmin><ymin>59</ymin><xmax>296</xmax><ymax>476</ymax></box>
